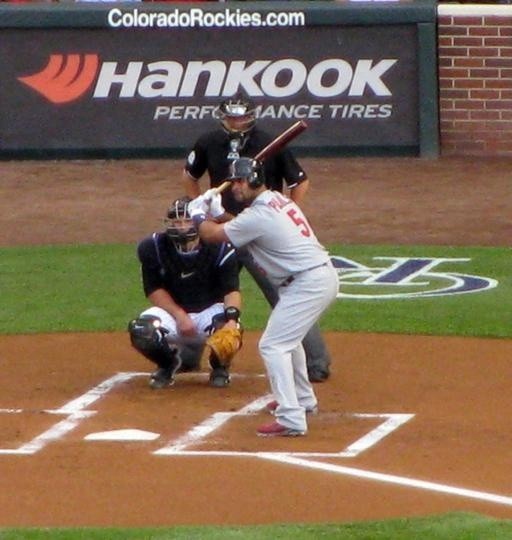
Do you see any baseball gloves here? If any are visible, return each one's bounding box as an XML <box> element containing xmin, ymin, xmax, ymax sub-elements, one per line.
<box><xmin>200</xmin><ymin>324</ymin><xmax>243</xmax><ymax>373</ymax></box>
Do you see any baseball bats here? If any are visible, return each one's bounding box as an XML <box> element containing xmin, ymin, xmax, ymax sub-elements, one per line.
<box><xmin>204</xmin><ymin>120</ymin><xmax>307</xmax><ymax>205</ymax></box>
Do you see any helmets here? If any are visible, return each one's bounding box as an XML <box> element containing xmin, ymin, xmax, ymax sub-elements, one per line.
<box><xmin>160</xmin><ymin>196</ymin><xmax>203</xmax><ymax>256</ymax></box>
<box><xmin>223</xmin><ymin>157</ymin><xmax>267</xmax><ymax>184</ymax></box>
<box><xmin>218</xmin><ymin>96</ymin><xmax>259</xmax><ymax>141</ymax></box>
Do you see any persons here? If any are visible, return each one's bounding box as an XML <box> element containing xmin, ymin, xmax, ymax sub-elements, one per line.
<box><xmin>173</xmin><ymin>96</ymin><xmax>334</xmax><ymax>382</ymax></box>
<box><xmin>126</xmin><ymin>196</ymin><xmax>242</xmax><ymax>391</ymax></box>
<box><xmin>186</xmin><ymin>157</ymin><xmax>340</xmax><ymax>436</ymax></box>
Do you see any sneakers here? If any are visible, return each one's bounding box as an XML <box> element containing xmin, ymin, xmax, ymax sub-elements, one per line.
<box><xmin>307</xmin><ymin>364</ymin><xmax>330</xmax><ymax>383</ymax></box>
<box><xmin>149</xmin><ymin>355</ymin><xmax>182</xmax><ymax>389</ymax></box>
<box><xmin>264</xmin><ymin>400</ymin><xmax>317</xmax><ymax>413</ymax></box>
<box><xmin>256</xmin><ymin>422</ymin><xmax>305</xmax><ymax>438</ymax></box>
<box><xmin>208</xmin><ymin>354</ymin><xmax>231</xmax><ymax>388</ymax></box>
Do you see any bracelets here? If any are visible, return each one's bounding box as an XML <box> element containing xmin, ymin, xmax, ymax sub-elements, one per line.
<box><xmin>225</xmin><ymin>305</ymin><xmax>240</xmax><ymax>323</ymax></box>
<box><xmin>193</xmin><ymin>214</ymin><xmax>209</xmax><ymax>232</ymax></box>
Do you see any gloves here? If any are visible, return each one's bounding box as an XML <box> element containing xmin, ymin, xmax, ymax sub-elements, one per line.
<box><xmin>187</xmin><ymin>188</ymin><xmax>226</xmax><ymax>229</ymax></box>
